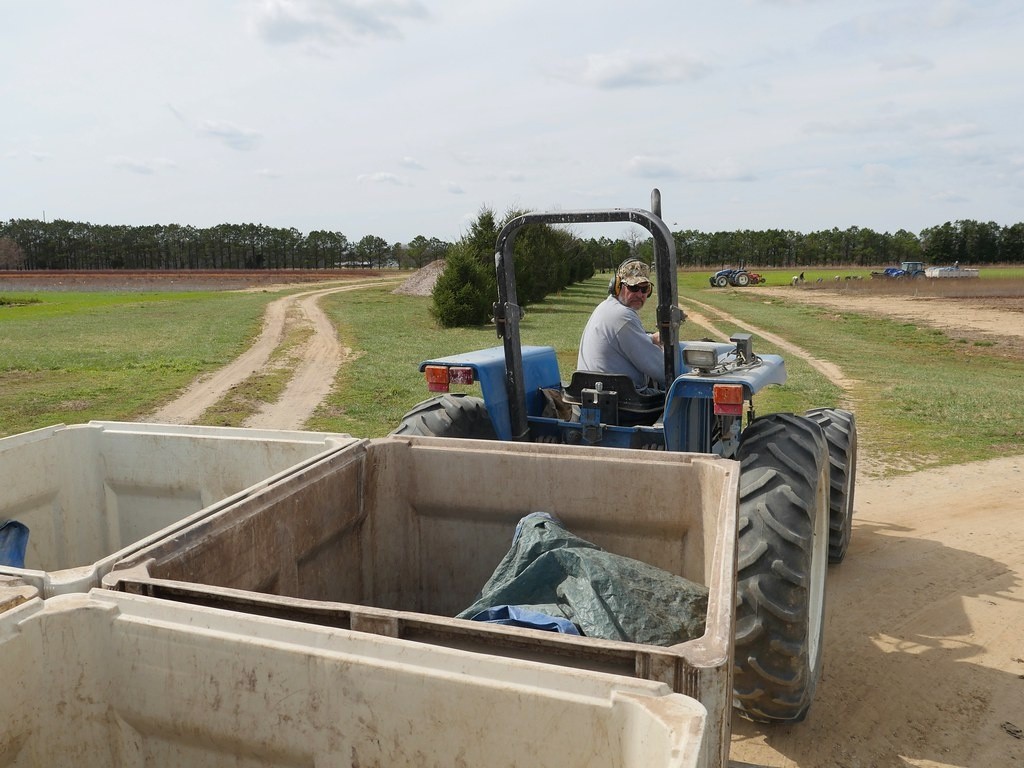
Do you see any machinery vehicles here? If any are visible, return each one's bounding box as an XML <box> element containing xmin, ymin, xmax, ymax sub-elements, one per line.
<box><xmin>884</xmin><ymin>261</ymin><xmax>979</xmax><ymax>281</ymax></box>
<box><xmin>708</xmin><ymin>258</ymin><xmax>765</xmax><ymax>288</ymax></box>
<box><xmin>0</xmin><ymin>187</ymin><xmax>859</xmax><ymax>767</ymax></box>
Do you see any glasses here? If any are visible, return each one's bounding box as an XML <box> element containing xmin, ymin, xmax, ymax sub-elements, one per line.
<box><xmin>622</xmin><ymin>283</ymin><xmax>651</xmax><ymax>293</ymax></box>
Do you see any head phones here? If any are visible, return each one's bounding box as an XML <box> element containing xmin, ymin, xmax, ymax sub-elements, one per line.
<box><xmin>607</xmin><ymin>259</ymin><xmax>652</xmax><ymax>298</ymax></box>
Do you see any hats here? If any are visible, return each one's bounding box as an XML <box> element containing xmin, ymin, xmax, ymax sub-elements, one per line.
<box><xmin>615</xmin><ymin>258</ymin><xmax>654</xmax><ymax>287</ymax></box>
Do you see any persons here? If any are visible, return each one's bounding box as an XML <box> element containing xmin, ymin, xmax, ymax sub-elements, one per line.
<box><xmin>791</xmin><ymin>271</ymin><xmax>862</xmax><ymax>283</ymax></box>
<box><xmin>954</xmin><ymin>260</ymin><xmax>958</xmax><ymax>268</ymax></box>
<box><xmin>576</xmin><ymin>260</ymin><xmax>666</xmax><ymax>396</ymax></box>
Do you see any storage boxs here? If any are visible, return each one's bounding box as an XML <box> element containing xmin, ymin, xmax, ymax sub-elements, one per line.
<box><xmin>0</xmin><ymin>421</ymin><xmax>743</xmax><ymax>768</ymax></box>
